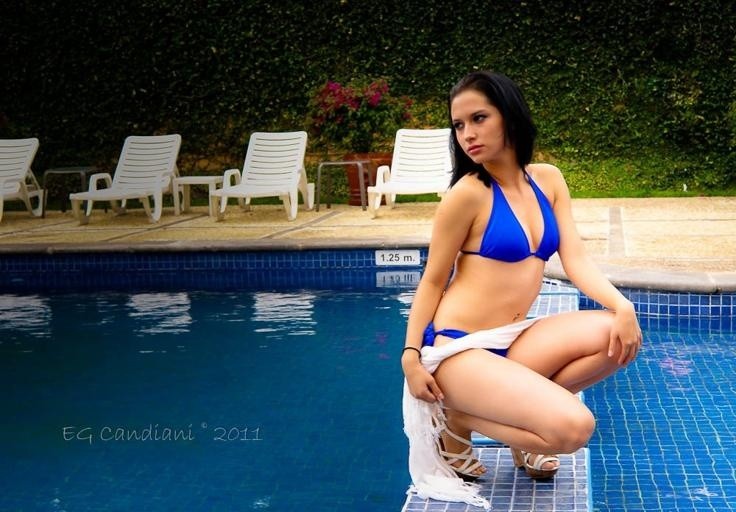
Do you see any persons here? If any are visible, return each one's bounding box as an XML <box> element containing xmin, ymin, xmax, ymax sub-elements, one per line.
<box><xmin>397</xmin><ymin>68</ymin><xmax>646</xmax><ymax>485</ymax></box>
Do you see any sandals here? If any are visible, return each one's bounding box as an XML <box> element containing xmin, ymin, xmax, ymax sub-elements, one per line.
<box><xmin>429</xmin><ymin>402</ymin><xmax>487</xmax><ymax>483</ymax></box>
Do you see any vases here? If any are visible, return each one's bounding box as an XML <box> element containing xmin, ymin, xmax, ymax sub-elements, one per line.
<box><xmin>343</xmin><ymin>152</ymin><xmax>392</xmax><ymax>206</ymax></box>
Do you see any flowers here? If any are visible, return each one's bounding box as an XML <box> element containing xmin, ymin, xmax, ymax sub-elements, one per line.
<box><xmin>302</xmin><ymin>77</ymin><xmax>414</xmax><ymax>153</ymax></box>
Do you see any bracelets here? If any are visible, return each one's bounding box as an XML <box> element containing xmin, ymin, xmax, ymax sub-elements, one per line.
<box><xmin>402</xmin><ymin>346</ymin><xmax>422</xmax><ymax>357</ymax></box>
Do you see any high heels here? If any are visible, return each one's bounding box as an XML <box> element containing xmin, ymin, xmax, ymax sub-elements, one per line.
<box><xmin>510</xmin><ymin>446</ymin><xmax>560</xmax><ymax>478</ymax></box>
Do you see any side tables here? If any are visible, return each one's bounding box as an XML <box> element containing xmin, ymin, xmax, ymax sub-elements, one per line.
<box><xmin>172</xmin><ymin>175</ymin><xmax>224</xmax><ymax>217</ymax></box>
<box><xmin>315</xmin><ymin>158</ymin><xmax>373</xmax><ymax>212</ymax></box>
<box><xmin>42</xmin><ymin>166</ymin><xmax>108</xmax><ymax>219</ymax></box>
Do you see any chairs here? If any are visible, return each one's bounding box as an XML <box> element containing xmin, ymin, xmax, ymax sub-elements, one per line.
<box><xmin>68</xmin><ymin>133</ymin><xmax>183</xmax><ymax>226</ymax></box>
<box><xmin>210</xmin><ymin>130</ymin><xmax>315</xmax><ymax>222</ymax></box>
<box><xmin>366</xmin><ymin>129</ymin><xmax>453</xmax><ymax>219</ymax></box>
<box><xmin>0</xmin><ymin>137</ymin><xmax>49</xmax><ymax>223</ymax></box>
